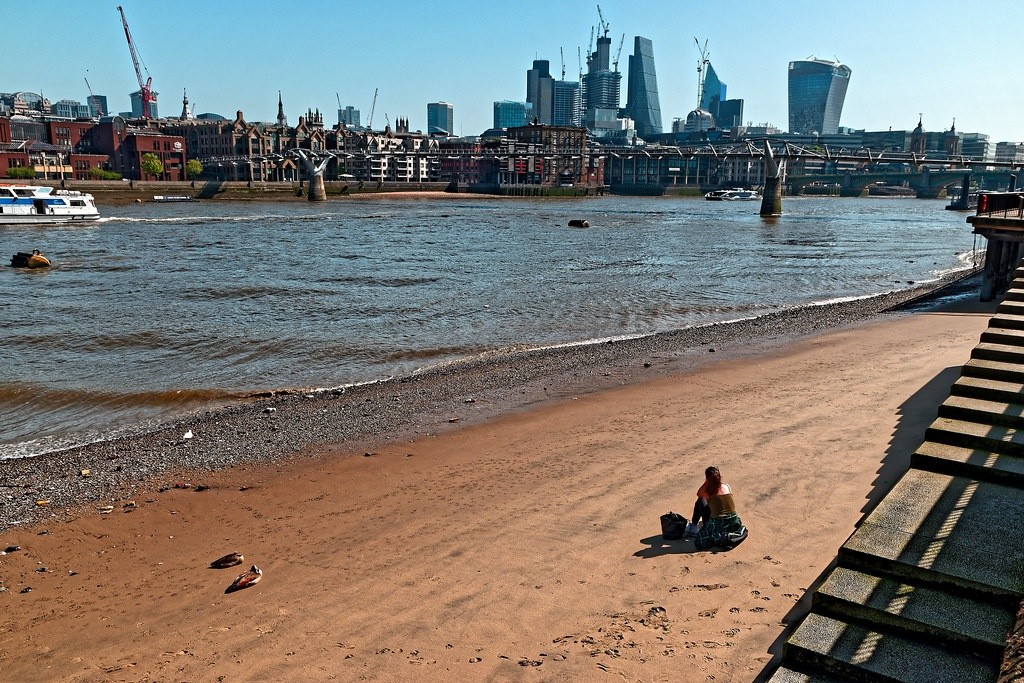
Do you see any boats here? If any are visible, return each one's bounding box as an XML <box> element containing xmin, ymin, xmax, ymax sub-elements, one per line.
<box><xmin>0</xmin><ymin>181</ymin><xmax>105</xmax><ymax>223</ymax></box>
<box><xmin>705</xmin><ymin>188</ymin><xmax>761</xmax><ymax>200</ymax></box>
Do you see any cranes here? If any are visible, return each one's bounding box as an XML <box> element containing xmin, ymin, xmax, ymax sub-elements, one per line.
<box><xmin>117</xmin><ymin>5</ymin><xmax>159</xmax><ymax>121</ymax></box>
<box><xmin>558</xmin><ymin>4</ymin><xmax>710</xmax><ymax>112</ymax></box>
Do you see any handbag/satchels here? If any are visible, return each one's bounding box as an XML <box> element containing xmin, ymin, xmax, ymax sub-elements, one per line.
<box><xmin>661</xmin><ymin>511</ymin><xmax>691</xmax><ymax>540</ymax></box>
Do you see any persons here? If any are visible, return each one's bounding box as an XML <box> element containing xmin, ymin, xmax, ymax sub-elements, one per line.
<box><xmin>686</xmin><ymin>466</ymin><xmax>741</xmax><ymax>536</ymax></box>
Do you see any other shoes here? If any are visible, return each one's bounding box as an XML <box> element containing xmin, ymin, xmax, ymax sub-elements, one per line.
<box><xmin>685</xmin><ymin>523</ymin><xmax>699</xmax><ymax>534</ymax></box>
